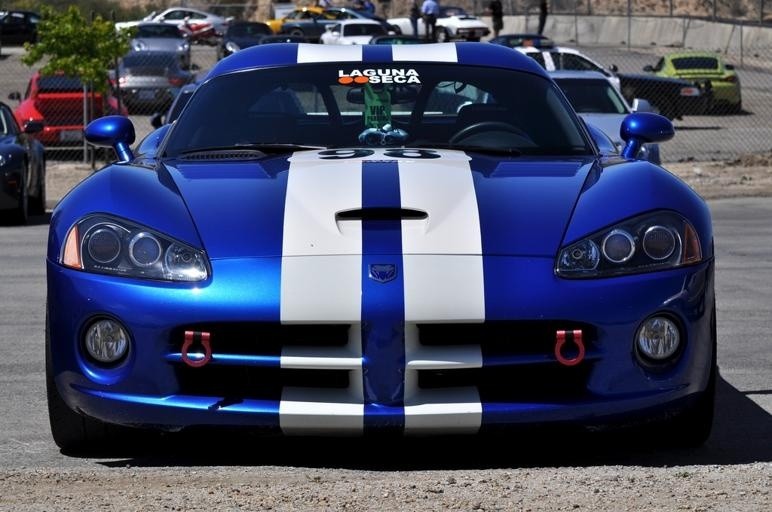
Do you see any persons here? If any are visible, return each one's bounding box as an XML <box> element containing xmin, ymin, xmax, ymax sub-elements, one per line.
<box><xmin>486</xmin><ymin>0</ymin><xmax>503</xmax><ymax>38</ymax></box>
<box><xmin>419</xmin><ymin>0</ymin><xmax>440</xmax><ymax>38</ymax></box>
<box><xmin>317</xmin><ymin>0</ymin><xmax>375</xmax><ymax>14</ymax></box>
<box><xmin>409</xmin><ymin>0</ymin><xmax>420</xmax><ymax>35</ymax></box>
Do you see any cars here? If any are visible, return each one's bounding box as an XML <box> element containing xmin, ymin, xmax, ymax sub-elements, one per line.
<box><xmin>10</xmin><ymin>68</ymin><xmax>128</xmax><ymax>148</ymax></box>
<box><xmin>124</xmin><ymin>20</ymin><xmax>191</xmax><ymax>68</ymax></box>
<box><xmin>481</xmin><ymin>33</ymin><xmax>718</xmax><ymax>122</ymax></box>
<box><xmin>114</xmin><ymin>5</ymin><xmax>236</xmax><ymax>46</ymax></box>
<box><xmin>108</xmin><ymin>50</ymin><xmax>204</xmax><ymax>116</ymax></box>
<box><xmin>458</xmin><ymin>70</ymin><xmax>653</xmax><ymax>161</ymax></box>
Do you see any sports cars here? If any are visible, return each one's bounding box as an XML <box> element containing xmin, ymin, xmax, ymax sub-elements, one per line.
<box><xmin>0</xmin><ymin>101</ymin><xmax>45</xmax><ymax>225</ymax></box>
<box><xmin>0</xmin><ymin>10</ymin><xmax>41</xmax><ymax>45</ymax></box>
<box><xmin>642</xmin><ymin>48</ymin><xmax>743</xmax><ymax>112</ymax></box>
<box><xmin>216</xmin><ymin>0</ymin><xmax>492</xmax><ymax>203</ymax></box>
<box><xmin>44</xmin><ymin>38</ymin><xmax>718</xmax><ymax>453</ymax></box>
